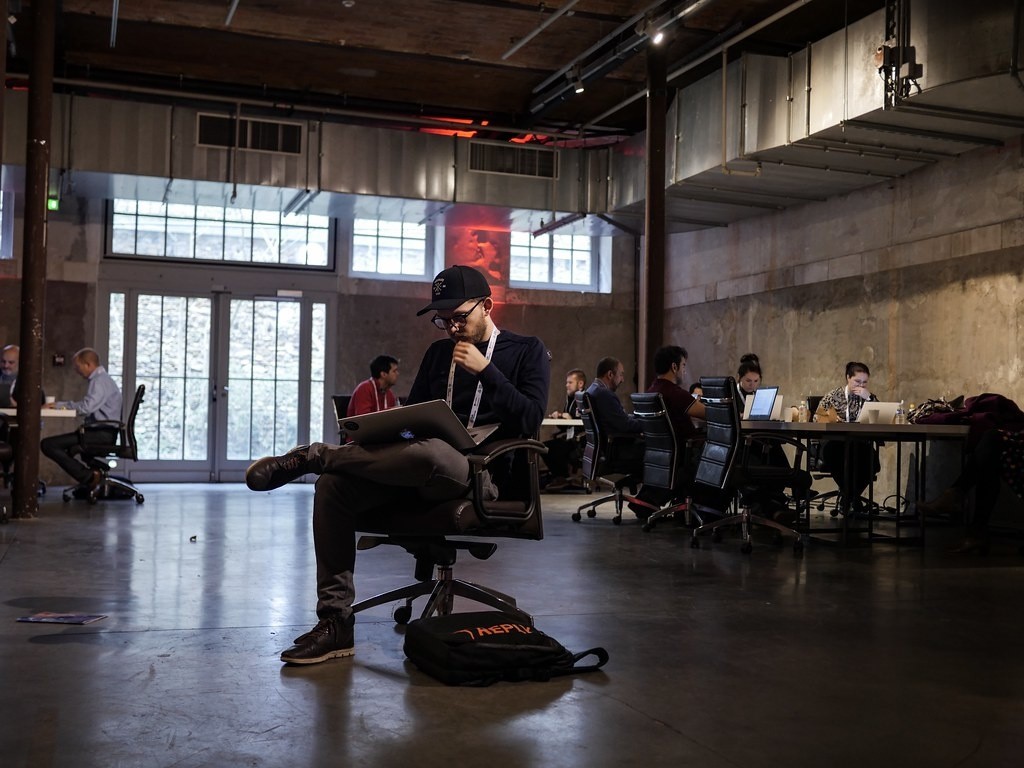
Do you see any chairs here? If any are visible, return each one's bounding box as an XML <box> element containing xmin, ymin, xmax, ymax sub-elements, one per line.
<box><xmin>631</xmin><ymin>394</ymin><xmax>732</xmax><ymax>546</ymax></box>
<box><xmin>346</xmin><ymin>438</ymin><xmax>550</xmax><ymax>622</ymax></box>
<box><xmin>689</xmin><ymin>374</ymin><xmax>808</xmax><ymax>562</ymax></box>
<box><xmin>61</xmin><ymin>382</ymin><xmax>147</xmax><ymax>505</ymax></box>
<box><xmin>331</xmin><ymin>393</ymin><xmax>352</xmax><ymax>444</ymax></box>
<box><xmin>571</xmin><ymin>390</ymin><xmax>657</xmax><ymax>525</ymax></box>
<box><xmin>0</xmin><ymin>388</ymin><xmax>47</xmax><ymax>495</ymax></box>
<box><xmin>807</xmin><ymin>393</ymin><xmax>886</xmax><ymax>517</ymax></box>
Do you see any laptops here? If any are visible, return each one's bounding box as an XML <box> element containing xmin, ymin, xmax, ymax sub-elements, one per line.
<box><xmin>855</xmin><ymin>401</ymin><xmax>899</xmax><ymax>424</ymax></box>
<box><xmin>742</xmin><ymin>395</ymin><xmax>783</xmax><ymax>420</ymax></box>
<box><xmin>740</xmin><ymin>386</ymin><xmax>779</xmax><ymax>420</ymax></box>
<box><xmin>336</xmin><ymin>398</ymin><xmax>503</xmax><ymax>452</ymax></box>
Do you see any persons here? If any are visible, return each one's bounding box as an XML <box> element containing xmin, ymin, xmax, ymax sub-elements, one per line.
<box><xmin>735</xmin><ymin>353</ymin><xmax>819</xmax><ymax>501</ymax></box>
<box><xmin>585</xmin><ymin>357</ymin><xmax>669</xmax><ymax>518</ymax></box>
<box><xmin>540</xmin><ymin>370</ymin><xmax>588</xmax><ymax>489</ymax></box>
<box><xmin>646</xmin><ymin>346</ymin><xmax>730</xmax><ymax>530</ymax></box>
<box><xmin>813</xmin><ymin>362</ymin><xmax>879</xmax><ymax>512</ymax></box>
<box><xmin>244</xmin><ymin>265</ymin><xmax>550</xmax><ymax>664</ymax></box>
<box><xmin>0</xmin><ymin>345</ymin><xmax>46</xmax><ymax>407</ymax></box>
<box><xmin>40</xmin><ymin>348</ymin><xmax>122</xmax><ymax>499</ymax></box>
<box><xmin>345</xmin><ymin>355</ymin><xmax>399</xmax><ymax>443</ymax></box>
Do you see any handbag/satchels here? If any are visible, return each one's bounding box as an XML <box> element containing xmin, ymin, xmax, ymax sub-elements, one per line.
<box><xmin>907</xmin><ymin>398</ymin><xmax>954</xmax><ymax>424</ymax></box>
<box><xmin>404</xmin><ymin>610</ymin><xmax>609</xmax><ymax>686</ymax></box>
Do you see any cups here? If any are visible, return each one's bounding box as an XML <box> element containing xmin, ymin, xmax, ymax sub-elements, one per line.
<box><xmin>45</xmin><ymin>396</ymin><xmax>55</xmax><ymax>404</ymax></box>
<box><xmin>868</xmin><ymin>409</ymin><xmax>880</xmax><ymax>424</ymax></box>
<box><xmin>783</xmin><ymin>408</ymin><xmax>793</xmax><ymax>423</ymax></box>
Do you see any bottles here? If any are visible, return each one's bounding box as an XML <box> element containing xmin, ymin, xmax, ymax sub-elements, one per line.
<box><xmin>908</xmin><ymin>405</ymin><xmax>915</xmax><ymax>425</ymax></box>
<box><xmin>895</xmin><ymin>404</ymin><xmax>906</xmax><ymax>424</ymax></box>
<box><xmin>798</xmin><ymin>401</ymin><xmax>807</xmax><ymax>423</ymax></box>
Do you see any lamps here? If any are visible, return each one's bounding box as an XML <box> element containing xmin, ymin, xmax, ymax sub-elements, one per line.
<box><xmin>565</xmin><ymin>65</ymin><xmax>585</xmax><ymax>93</ymax></box>
<box><xmin>635</xmin><ymin>10</ymin><xmax>665</xmax><ymax>44</ymax></box>
<box><xmin>534</xmin><ymin>212</ymin><xmax>587</xmax><ymax>238</ymax></box>
<box><xmin>283</xmin><ymin>188</ymin><xmax>310</xmax><ymax>218</ymax></box>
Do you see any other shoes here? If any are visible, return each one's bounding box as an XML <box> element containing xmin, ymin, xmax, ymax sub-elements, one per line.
<box><xmin>793</xmin><ymin>490</ymin><xmax>818</xmax><ymax>502</ymax></box>
<box><xmin>838</xmin><ymin>494</ymin><xmax>850</xmax><ymax>515</ymax></box>
<box><xmin>88</xmin><ymin>470</ymin><xmax>101</xmax><ymax>498</ymax></box>
<box><xmin>852</xmin><ymin>493</ymin><xmax>863</xmax><ymax>512</ymax></box>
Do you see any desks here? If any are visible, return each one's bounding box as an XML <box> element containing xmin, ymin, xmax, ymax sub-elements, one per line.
<box><xmin>1</xmin><ymin>407</ymin><xmax>78</xmax><ymax>417</ymax></box>
<box><xmin>539</xmin><ymin>418</ymin><xmax>585</xmax><ymax>426</ymax></box>
<box><xmin>693</xmin><ymin>422</ymin><xmax>975</xmax><ymax>548</ymax></box>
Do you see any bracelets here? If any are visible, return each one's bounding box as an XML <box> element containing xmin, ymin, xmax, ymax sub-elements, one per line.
<box><xmin>867</xmin><ymin>394</ymin><xmax>875</xmax><ymax>401</ymax></box>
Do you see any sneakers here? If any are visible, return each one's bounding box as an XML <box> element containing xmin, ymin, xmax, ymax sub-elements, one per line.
<box><xmin>566</xmin><ymin>466</ymin><xmax>583</xmax><ymax>487</ymax></box>
<box><xmin>280</xmin><ymin>611</ymin><xmax>355</xmax><ymax>664</ymax></box>
<box><xmin>545</xmin><ymin>474</ymin><xmax>568</xmax><ymax>490</ymax></box>
<box><xmin>246</xmin><ymin>445</ymin><xmax>324</xmax><ymax>491</ymax></box>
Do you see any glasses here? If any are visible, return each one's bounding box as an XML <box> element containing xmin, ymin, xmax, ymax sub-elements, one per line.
<box><xmin>431</xmin><ymin>296</ymin><xmax>485</xmax><ymax>330</ymax></box>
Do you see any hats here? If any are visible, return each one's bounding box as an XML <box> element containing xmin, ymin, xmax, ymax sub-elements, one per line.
<box><xmin>416</xmin><ymin>265</ymin><xmax>491</xmax><ymax>316</ymax></box>
